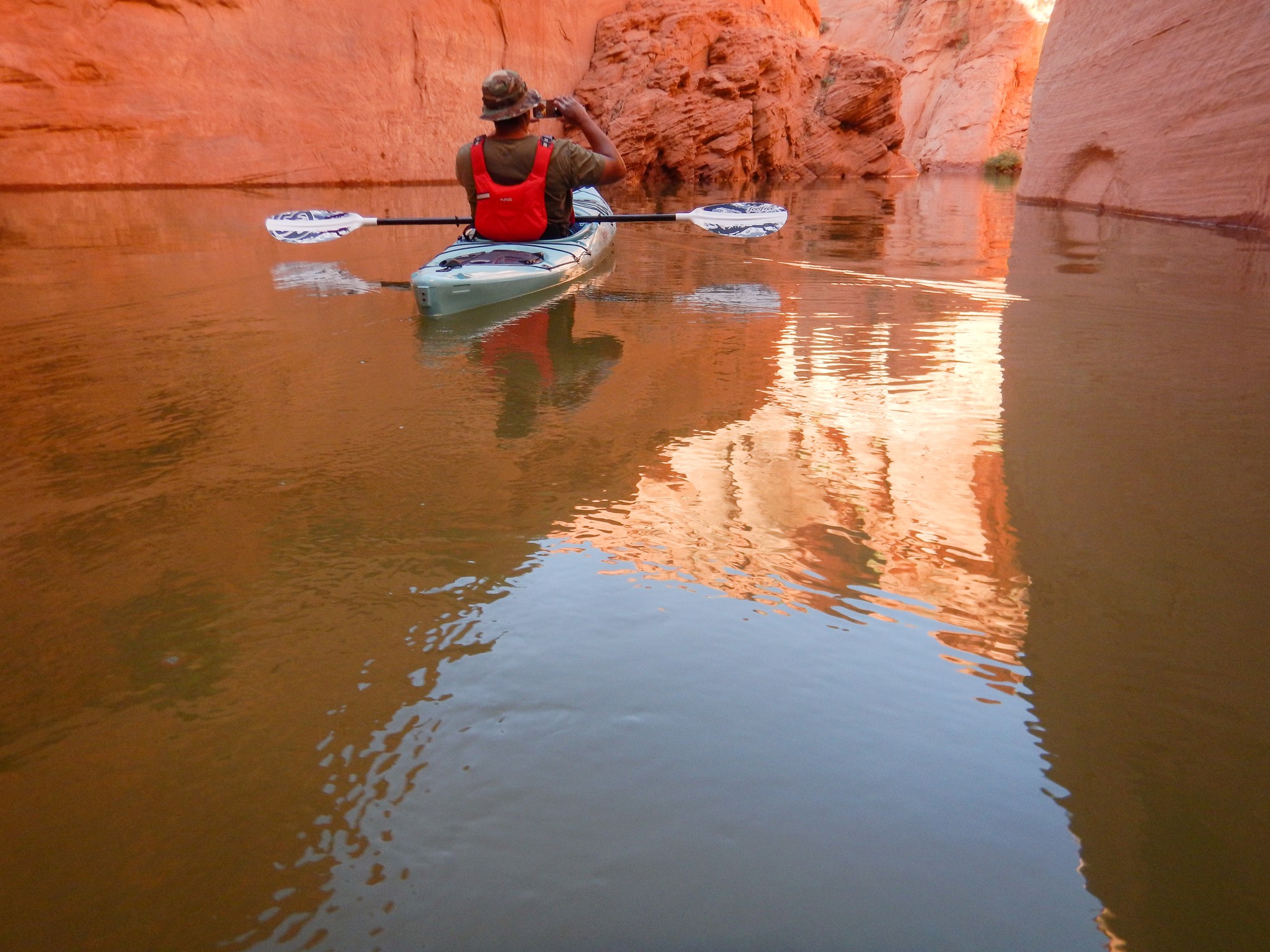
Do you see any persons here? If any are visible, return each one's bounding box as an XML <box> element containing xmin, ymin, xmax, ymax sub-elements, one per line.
<box><xmin>454</xmin><ymin>70</ymin><xmax>627</xmax><ymax>242</ymax></box>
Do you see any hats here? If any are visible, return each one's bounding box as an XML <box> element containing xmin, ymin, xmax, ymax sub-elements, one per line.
<box><xmin>478</xmin><ymin>71</ymin><xmax>541</xmax><ymax>122</ymax></box>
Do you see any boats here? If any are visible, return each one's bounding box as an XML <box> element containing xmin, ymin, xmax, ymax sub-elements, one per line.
<box><xmin>409</xmin><ymin>186</ymin><xmax>618</xmax><ymax>317</ymax></box>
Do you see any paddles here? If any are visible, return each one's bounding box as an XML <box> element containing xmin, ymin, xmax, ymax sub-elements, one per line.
<box><xmin>271</xmin><ymin>259</ymin><xmax>780</xmax><ymax>317</ymax></box>
<box><xmin>265</xmin><ymin>201</ymin><xmax>789</xmax><ymax>245</ymax></box>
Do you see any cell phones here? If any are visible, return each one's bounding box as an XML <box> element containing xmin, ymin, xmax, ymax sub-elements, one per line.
<box><xmin>533</xmin><ymin>100</ymin><xmax>563</xmax><ymax>119</ymax></box>
